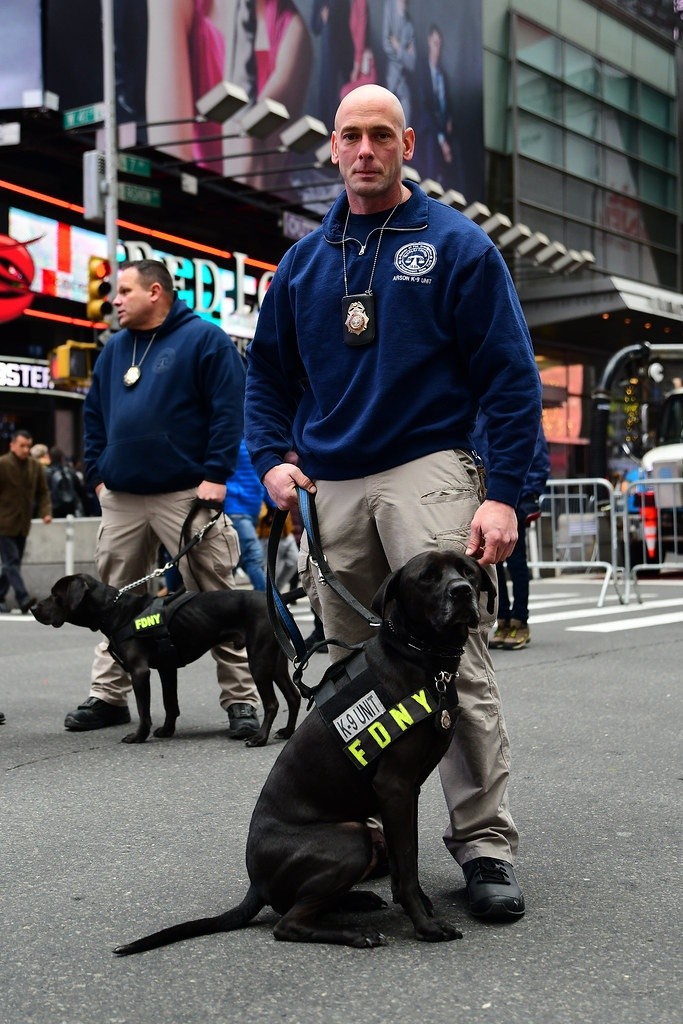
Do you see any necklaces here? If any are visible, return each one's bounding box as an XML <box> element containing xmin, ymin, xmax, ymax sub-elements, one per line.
<box><xmin>342</xmin><ymin>184</ymin><xmax>404</xmax><ymax>345</ymax></box>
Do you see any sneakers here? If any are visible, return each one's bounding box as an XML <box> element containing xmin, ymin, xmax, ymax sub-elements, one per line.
<box><xmin>62</xmin><ymin>694</ymin><xmax>131</xmax><ymax>732</ymax></box>
<box><xmin>461</xmin><ymin>850</ymin><xmax>526</xmax><ymax>917</ymax></box>
<box><xmin>226</xmin><ymin>700</ymin><xmax>260</xmax><ymax>740</ymax></box>
<box><xmin>489</xmin><ymin>617</ymin><xmax>532</xmax><ymax>649</ymax></box>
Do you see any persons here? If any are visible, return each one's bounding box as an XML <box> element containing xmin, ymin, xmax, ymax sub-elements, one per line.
<box><xmin>172</xmin><ymin>2</ymin><xmax>460</xmax><ymax>224</ymax></box>
<box><xmin>0</xmin><ymin>430</ymin><xmax>84</xmax><ymax>614</ymax></box>
<box><xmin>66</xmin><ymin>82</ymin><xmax>552</xmax><ymax>924</ymax></box>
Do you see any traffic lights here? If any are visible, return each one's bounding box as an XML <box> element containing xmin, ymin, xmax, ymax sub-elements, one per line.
<box><xmin>54</xmin><ymin>344</ymin><xmax>91</xmax><ymax>381</ymax></box>
<box><xmin>87</xmin><ymin>254</ymin><xmax>114</xmax><ymax>321</ymax></box>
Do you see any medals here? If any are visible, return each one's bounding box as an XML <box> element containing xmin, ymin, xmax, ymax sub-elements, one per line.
<box><xmin>122</xmin><ymin>365</ymin><xmax>142</xmax><ymax>387</ymax></box>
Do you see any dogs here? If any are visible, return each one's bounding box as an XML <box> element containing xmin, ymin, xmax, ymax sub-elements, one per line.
<box><xmin>29</xmin><ymin>571</ymin><xmax>310</xmax><ymax>751</ymax></box>
<box><xmin>111</xmin><ymin>546</ymin><xmax>501</xmax><ymax>958</ymax></box>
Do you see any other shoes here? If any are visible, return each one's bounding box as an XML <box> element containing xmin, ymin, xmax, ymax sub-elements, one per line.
<box><xmin>18</xmin><ymin>596</ymin><xmax>37</xmax><ymax>614</ymax></box>
<box><xmin>0</xmin><ymin>597</ymin><xmax>11</xmax><ymax>612</ymax></box>
